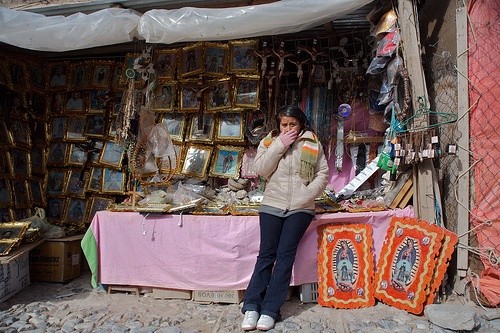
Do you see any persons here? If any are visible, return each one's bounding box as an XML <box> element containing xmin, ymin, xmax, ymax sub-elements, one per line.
<box><xmin>241</xmin><ymin>104</ymin><xmax>330</xmax><ymax>331</ymax></box>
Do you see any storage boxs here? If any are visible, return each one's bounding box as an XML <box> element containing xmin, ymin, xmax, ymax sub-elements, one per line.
<box><xmin>0</xmin><ymin>256</ymin><xmax>31</xmax><ymax>303</ymax></box>
<box><xmin>32</xmin><ymin>243</ymin><xmax>81</xmax><ymax>283</ymax></box>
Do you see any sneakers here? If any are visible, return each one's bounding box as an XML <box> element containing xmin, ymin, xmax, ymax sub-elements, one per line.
<box><xmin>241</xmin><ymin>310</ymin><xmax>259</xmax><ymax>330</ymax></box>
<box><xmin>256</xmin><ymin>315</ymin><xmax>275</xmax><ymax>330</ymax></box>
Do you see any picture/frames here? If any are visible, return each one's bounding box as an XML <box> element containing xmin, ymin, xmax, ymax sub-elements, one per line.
<box><xmin>0</xmin><ymin>37</ymin><xmax>261</xmax><ymax>256</ymax></box>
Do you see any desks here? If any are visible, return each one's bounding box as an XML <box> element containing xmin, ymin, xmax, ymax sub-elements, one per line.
<box><xmin>81</xmin><ymin>210</ymin><xmax>415</xmax><ymax>304</ymax></box>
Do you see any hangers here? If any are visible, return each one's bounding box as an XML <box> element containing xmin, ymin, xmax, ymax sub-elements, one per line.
<box><xmin>394</xmin><ymin>96</ymin><xmax>458</xmax><ymax>134</ymax></box>
